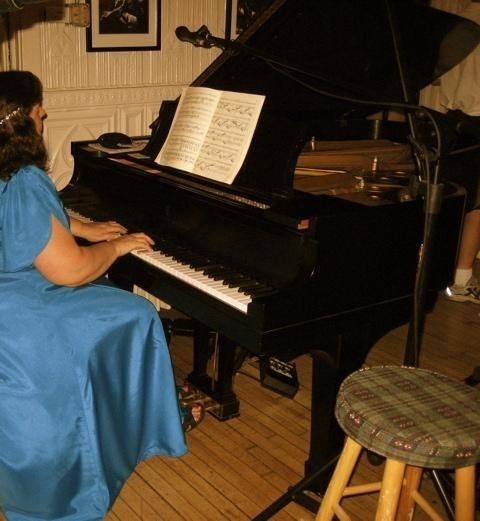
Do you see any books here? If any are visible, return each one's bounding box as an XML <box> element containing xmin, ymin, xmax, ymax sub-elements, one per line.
<box><xmin>153</xmin><ymin>84</ymin><xmax>266</xmax><ymax>186</ymax></box>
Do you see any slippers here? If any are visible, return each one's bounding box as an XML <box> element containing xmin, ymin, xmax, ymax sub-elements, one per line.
<box><xmin>179</xmin><ymin>402</ymin><xmax>205</xmax><ymax>432</ymax></box>
<box><xmin>176</xmin><ymin>382</ymin><xmax>193</xmax><ymax>400</ymax></box>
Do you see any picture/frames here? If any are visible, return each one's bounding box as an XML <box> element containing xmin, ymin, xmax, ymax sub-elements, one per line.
<box><xmin>84</xmin><ymin>0</ymin><xmax>161</xmax><ymax>53</ymax></box>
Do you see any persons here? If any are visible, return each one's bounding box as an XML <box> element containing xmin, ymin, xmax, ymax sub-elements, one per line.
<box><xmin>428</xmin><ymin>0</ymin><xmax>480</xmax><ymax>304</ymax></box>
<box><xmin>0</xmin><ymin>69</ymin><xmax>207</xmax><ymax>521</ymax></box>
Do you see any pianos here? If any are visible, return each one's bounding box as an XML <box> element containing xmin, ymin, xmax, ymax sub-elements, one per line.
<box><xmin>59</xmin><ymin>1</ymin><xmax>476</xmax><ymax>504</ymax></box>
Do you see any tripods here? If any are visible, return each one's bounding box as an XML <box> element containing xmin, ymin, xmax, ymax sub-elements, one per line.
<box><xmin>213</xmin><ymin>37</ymin><xmax>466</xmax><ymax>521</ymax></box>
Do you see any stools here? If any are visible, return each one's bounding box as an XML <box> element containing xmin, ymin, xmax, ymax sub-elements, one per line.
<box><xmin>314</xmin><ymin>360</ymin><xmax>480</xmax><ymax>521</ymax></box>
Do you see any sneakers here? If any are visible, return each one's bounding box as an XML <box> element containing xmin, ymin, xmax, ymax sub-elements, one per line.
<box><xmin>446</xmin><ymin>277</ymin><xmax>480</xmax><ymax>304</ymax></box>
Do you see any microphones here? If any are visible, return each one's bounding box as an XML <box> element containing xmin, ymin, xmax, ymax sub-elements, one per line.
<box><xmin>174</xmin><ymin>23</ymin><xmax>212</xmax><ymax>51</ymax></box>
